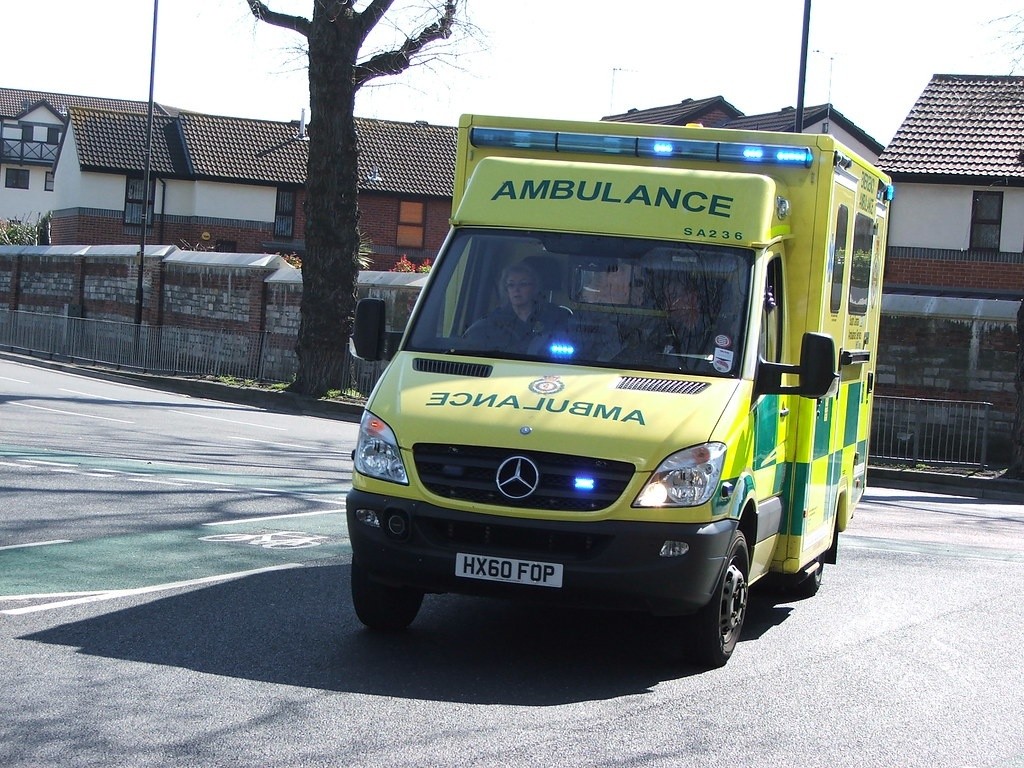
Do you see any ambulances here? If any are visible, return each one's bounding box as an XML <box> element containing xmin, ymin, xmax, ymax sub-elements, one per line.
<box><xmin>343</xmin><ymin>109</ymin><xmax>895</xmax><ymax>671</ymax></box>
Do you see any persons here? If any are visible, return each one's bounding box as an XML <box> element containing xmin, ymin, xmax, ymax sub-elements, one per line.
<box><xmin>647</xmin><ymin>273</ymin><xmax>727</xmax><ymax>375</ymax></box>
<box><xmin>471</xmin><ymin>258</ymin><xmax>576</xmax><ymax>359</ymax></box>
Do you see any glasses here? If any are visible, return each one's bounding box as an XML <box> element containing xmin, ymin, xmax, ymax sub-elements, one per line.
<box><xmin>504</xmin><ymin>282</ymin><xmax>535</xmax><ymax>288</ymax></box>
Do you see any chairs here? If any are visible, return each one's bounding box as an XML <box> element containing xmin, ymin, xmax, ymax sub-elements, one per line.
<box><xmin>523</xmin><ymin>255</ymin><xmax>576</xmax><ymax>334</ymax></box>
<box><xmin>696</xmin><ymin>277</ymin><xmax>733</xmax><ymax>335</ymax></box>
